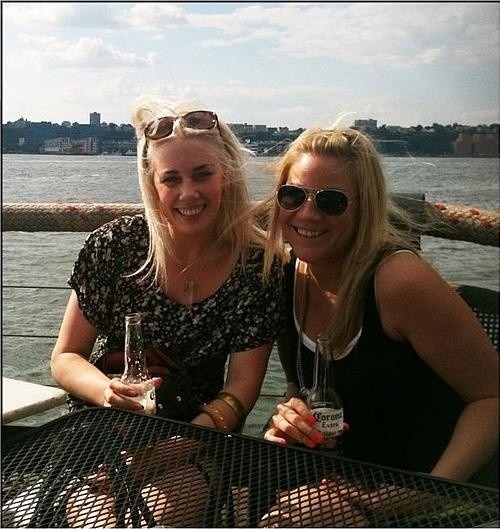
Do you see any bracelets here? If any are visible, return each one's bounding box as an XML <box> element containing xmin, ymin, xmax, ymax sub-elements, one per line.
<box><xmin>215</xmin><ymin>390</ymin><xmax>246</xmax><ymax>434</ymax></box>
<box><xmin>199</xmin><ymin>402</ymin><xmax>231</xmax><ymax>434</ymax></box>
<box><xmin>285</xmin><ymin>391</ymin><xmax>304</xmax><ymax>402</ymax></box>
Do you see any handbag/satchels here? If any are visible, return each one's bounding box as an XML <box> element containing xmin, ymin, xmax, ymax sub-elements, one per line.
<box><xmin>67</xmin><ymin>348</ymin><xmax>221</xmax><ymax>447</ymax></box>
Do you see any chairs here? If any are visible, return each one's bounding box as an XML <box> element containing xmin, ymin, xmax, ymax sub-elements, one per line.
<box><xmin>455</xmin><ymin>283</ymin><xmax>500</xmax><ymax>357</ymax></box>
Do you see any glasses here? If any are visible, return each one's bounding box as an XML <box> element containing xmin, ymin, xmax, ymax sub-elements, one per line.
<box><xmin>144</xmin><ymin>110</ymin><xmax>222</xmax><ymax>140</ymax></box>
<box><xmin>276</xmin><ymin>184</ymin><xmax>352</xmax><ymax>216</ymax></box>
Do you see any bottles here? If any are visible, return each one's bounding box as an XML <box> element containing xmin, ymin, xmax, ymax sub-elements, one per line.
<box><xmin>306</xmin><ymin>332</ymin><xmax>346</xmax><ymax>471</ymax></box>
<box><xmin>118</xmin><ymin>311</ymin><xmax>158</xmax><ymax>415</ymax></box>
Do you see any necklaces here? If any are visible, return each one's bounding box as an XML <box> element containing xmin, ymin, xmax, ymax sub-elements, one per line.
<box><xmin>296</xmin><ymin>263</ymin><xmax>309</xmax><ymax>391</ymax></box>
<box><xmin>183</xmin><ymin>276</ymin><xmax>196</xmax><ymax>292</ymax></box>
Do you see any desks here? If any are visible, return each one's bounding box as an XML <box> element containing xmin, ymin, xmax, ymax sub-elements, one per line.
<box><xmin>1</xmin><ymin>406</ymin><xmax>500</xmax><ymax>524</ymax></box>
<box><xmin>3</xmin><ymin>376</ymin><xmax>70</xmax><ymax>424</ymax></box>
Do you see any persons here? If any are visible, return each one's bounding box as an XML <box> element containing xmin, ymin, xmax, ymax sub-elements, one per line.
<box><xmin>0</xmin><ymin>98</ymin><xmax>287</xmax><ymax>528</ymax></box>
<box><xmin>253</xmin><ymin>125</ymin><xmax>500</xmax><ymax>529</ymax></box>
